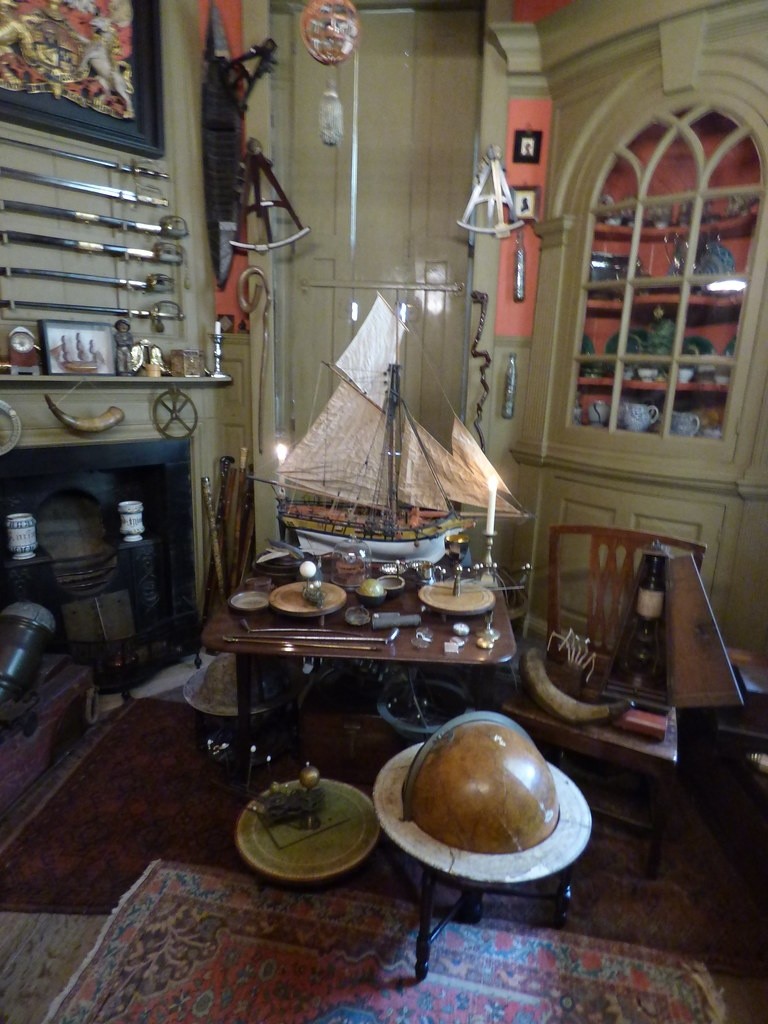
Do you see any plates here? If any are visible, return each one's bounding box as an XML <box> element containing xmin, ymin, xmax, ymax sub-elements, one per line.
<box><xmin>372</xmin><ymin>741</ymin><xmax>593</xmax><ymax>883</ymax></box>
<box><xmin>234</xmin><ymin>779</ymin><xmax>379</xmax><ymax>882</ymax></box>
<box><xmin>228</xmin><ymin>589</ymin><xmax>270</xmax><ymax>614</ymax></box>
<box><xmin>183</xmin><ymin>666</ymin><xmax>309</xmax><ymax>717</ymax></box>
<box><xmin>665</xmin><ymin>246</ymin><xmax>734</xmax><ymax>277</ymax></box>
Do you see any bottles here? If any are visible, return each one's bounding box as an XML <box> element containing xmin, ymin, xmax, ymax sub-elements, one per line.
<box><xmin>106</xmin><ymin>648</ymin><xmax>140</xmax><ymax>682</ymax></box>
<box><xmin>589</xmin><ymin>399</ymin><xmax>609</xmax><ymax>427</ymax></box>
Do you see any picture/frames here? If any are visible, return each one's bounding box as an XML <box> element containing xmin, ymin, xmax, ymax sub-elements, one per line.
<box><xmin>0</xmin><ymin>0</ymin><xmax>166</xmax><ymax>156</ymax></box>
<box><xmin>40</xmin><ymin>317</ymin><xmax>116</xmax><ymax>378</ymax></box>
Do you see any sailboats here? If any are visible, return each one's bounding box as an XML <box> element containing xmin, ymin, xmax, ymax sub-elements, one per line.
<box><xmin>241</xmin><ymin>283</ymin><xmax>537</xmax><ymax>569</ymax></box>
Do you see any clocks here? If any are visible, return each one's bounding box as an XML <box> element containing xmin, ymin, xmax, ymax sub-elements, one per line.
<box><xmin>8</xmin><ymin>327</ymin><xmax>40</xmax><ymax>375</ymax></box>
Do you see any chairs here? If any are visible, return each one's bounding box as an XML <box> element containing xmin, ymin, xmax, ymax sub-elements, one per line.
<box><xmin>502</xmin><ymin>524</ymin><xmax>706</xmax><ymax>878</ymax></box>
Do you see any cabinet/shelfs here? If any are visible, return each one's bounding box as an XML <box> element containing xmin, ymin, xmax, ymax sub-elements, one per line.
<box><xmin>499</xmin><ymin>0</ymin><xmax>768</xmax><ymax>686</ymax></box>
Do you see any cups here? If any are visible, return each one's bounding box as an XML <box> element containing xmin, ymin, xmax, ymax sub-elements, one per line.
<box><xmin>7</xmin><ymin>513</ymin><xmax>38</xmax><ymax>560</ymax></box>
<box><xmin>117</xmin><ymin>501</ymin><xmax>145</xmax><ymax>542</ymax></box>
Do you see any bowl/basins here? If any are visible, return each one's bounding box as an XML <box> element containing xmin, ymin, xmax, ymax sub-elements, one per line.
<box><xmin>677</xmin><ymin>369</ymin><xmax>694</xmax><ymax>384</ymax></box>
<box><xmin>637</xmin><ymin>367</ymin><xmax>658</xmax><ymax>382</ymax></box>
<box><xmin>378</xmin><ymin>680</ymin><xmax>477</xmax><ymax>751</ymax></box>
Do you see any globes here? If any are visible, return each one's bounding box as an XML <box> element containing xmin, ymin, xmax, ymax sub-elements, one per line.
<box><xmin>370</xmin><ymin>709</ymin><xmax>595</xmax><ymax>981</ymax></box>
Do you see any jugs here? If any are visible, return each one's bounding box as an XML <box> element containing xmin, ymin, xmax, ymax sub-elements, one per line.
<box><xmin>623</xmin><ymin>403</ymin><xmax>658</xmax><ymax>432</ymax></box>
<box><xmin>671</xmin><ymin>411</ymin><xmax>700</xmax><ymax>437</ymax></box>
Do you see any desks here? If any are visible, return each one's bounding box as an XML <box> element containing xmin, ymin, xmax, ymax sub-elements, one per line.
<box><xmin>198</xmin><ymin>555</ymin><xmax>516</xmax><ymax>787</ymax></box>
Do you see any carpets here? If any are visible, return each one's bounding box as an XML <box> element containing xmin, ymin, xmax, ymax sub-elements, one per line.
<box><xmin>40</xmin><ymin>860</ymin><xmax>723</xmax><ymax>1024</ymax></box>
